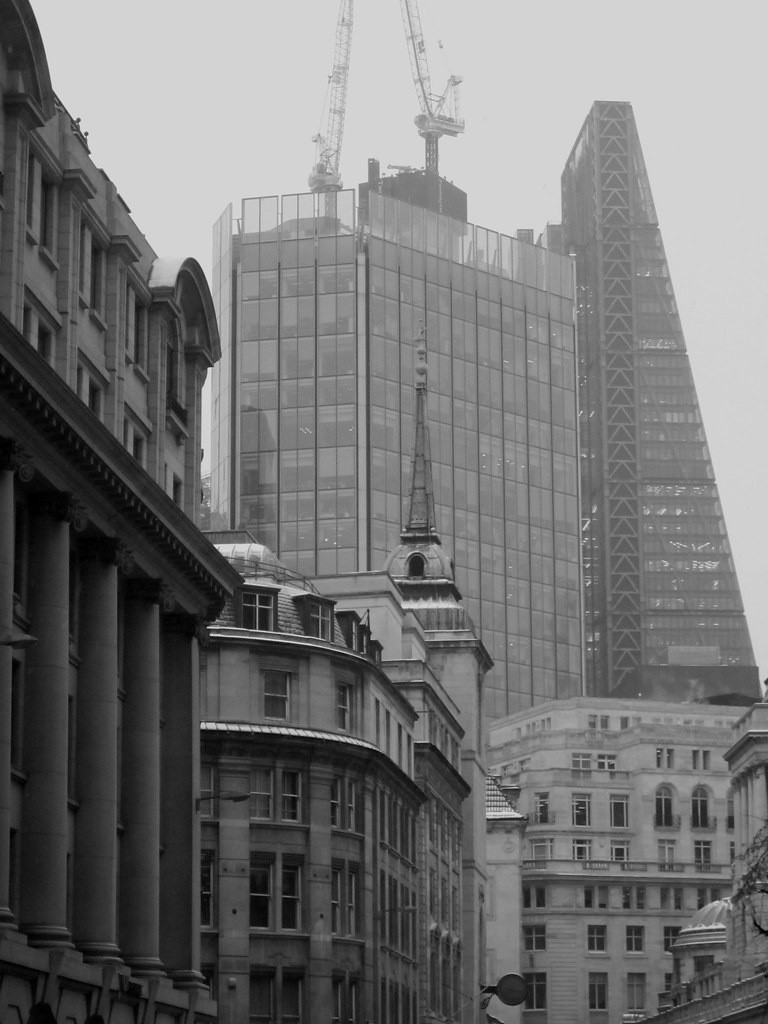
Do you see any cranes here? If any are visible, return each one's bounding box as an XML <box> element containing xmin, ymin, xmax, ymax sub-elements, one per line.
<box><xmin>394</xmin><ymin>0</ymin><xmax>467</xmax><ymax>222</ymax></box>
<box><xmin>310</xmin><ymin>2</ymin><xmax>356</xmax><ymax>226</ymax></box>
